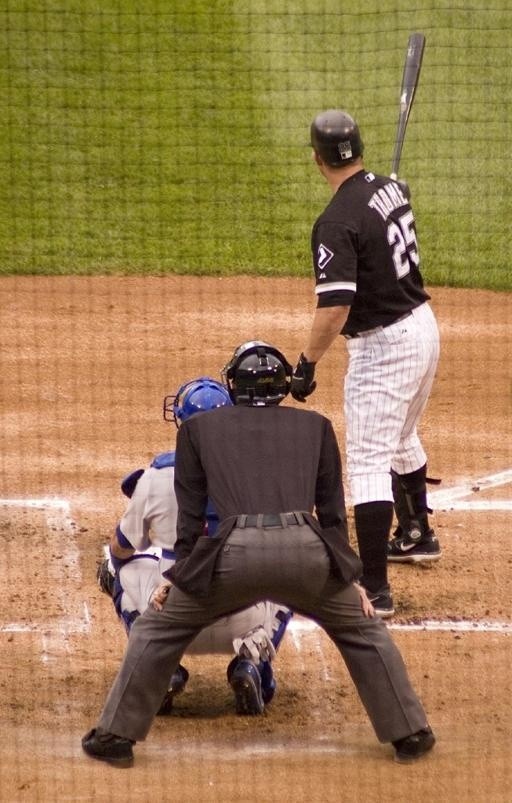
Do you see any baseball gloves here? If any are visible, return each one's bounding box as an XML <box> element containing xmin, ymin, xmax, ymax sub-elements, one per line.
<box><xmin>95</xmin><ymin>560</ymin><xmax>114</xmax><ymax>597</ymax></box>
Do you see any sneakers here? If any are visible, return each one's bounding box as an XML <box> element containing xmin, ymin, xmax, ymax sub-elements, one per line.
<box><xmin>391</xmin><ymin>725</ymin><xmax>437</xmax><ymax>765</ymax></box>
<box><xmin>155</xmin><ymin>668</ymin><xmax>186</xmax><ymax>717</ymax></box>
<box><xmin>365</xmin><ymin>583</ymin><xmax>395</xmax><ymax>618</ymax></box>
<box><xmin>229</xmin><ymin>659</ymin><xmax>265</xmax><ymax>716</ymax></box>
<box><xmin>386</xmin><ymin>529</ymin><xmax>442</xmax><ymax>564</ymax></box>
<box><xmin>81</xmin><ymin>728</ymin><xmax>135</xmax><ymax>769</ymax></box>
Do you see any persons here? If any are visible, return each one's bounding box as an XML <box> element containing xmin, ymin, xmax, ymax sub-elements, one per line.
<box><xmin>96</xmin><ymin>377</ymin><xmax>293</xmax><ymax>716</ymax></box>
<box><xmin>82</xmin><ymin>340</ymin><xmax>434</xmax><ymax>771</ymax></box>
<box><xmin>289</xmin><ymin>109</ymin><xmax>440</xmax><ymax>617</ymax></box>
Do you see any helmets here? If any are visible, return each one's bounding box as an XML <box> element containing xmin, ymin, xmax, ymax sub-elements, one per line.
<box><xmin>310</xmin><ymin>110</ymin><xmax>365</xmax><ymax>170</ymax></box>
<box><xmin>163</xmin><ymin>377</ymin><xmax>234</xmax><ymax>430</ymax></box>
<box><xmin>219</xmin><ymin>340</ymin><xmax>295</xmax><ymax>408</ymax></box>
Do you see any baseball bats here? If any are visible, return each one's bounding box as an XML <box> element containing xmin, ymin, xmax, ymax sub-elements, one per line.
<box><xmin>390</xmin><ymin>33</ymin><xmax>425</xmax><ymax>183</ymax></box>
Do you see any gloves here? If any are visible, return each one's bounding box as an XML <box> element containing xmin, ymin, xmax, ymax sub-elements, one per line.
<box><xmin>288</xmin><ymin>352</ymin><xmax>317</xmax><ymax>403</ymax></box>
